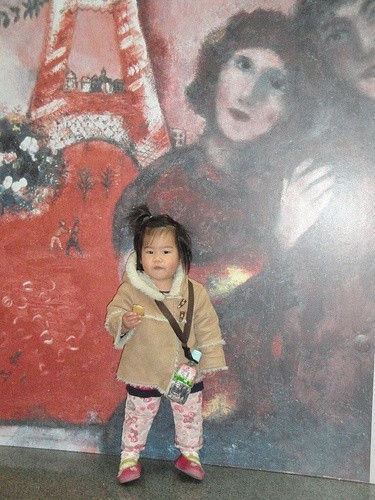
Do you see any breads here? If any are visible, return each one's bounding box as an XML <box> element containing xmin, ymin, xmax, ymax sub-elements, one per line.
<box><xmin>132</xmin><ymin>304</ymin><xmax>145</xmax><ymax>316</ymax></box>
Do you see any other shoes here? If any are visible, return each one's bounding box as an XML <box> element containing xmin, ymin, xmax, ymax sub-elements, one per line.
<box><xmin>118</xmin><ymin>460</ymin><xmax>140</xmax><ymax>483</ymax></box>
<box><xmin>174</xmin><ymin>453</ymin><xmax>205</xmax><ymax>480</ymax></box>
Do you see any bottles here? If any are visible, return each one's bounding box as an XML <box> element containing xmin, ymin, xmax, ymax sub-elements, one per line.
<box><xmin>165</xmin><ymin>350</ymin><xmax>203</xmax><ymax>405</ymax></box>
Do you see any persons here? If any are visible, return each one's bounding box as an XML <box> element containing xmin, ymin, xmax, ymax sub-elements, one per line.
<box><xmin>104</xmin><ymin>204</ymin><xmax>229</xmax><ymax>482</ymax></box>
